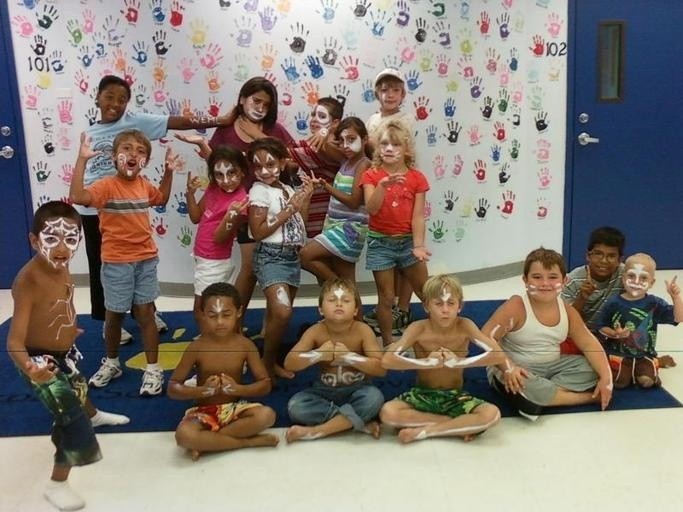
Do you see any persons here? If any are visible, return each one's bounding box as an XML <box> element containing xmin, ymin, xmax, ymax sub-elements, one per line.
<box><xmin>7</xmin><ymin>201</ymin><xmax>130</xmax><ymax>511</ymax></box>
<box><xmin>481</xmin><ymin>247</ymin><xmax>614</xmax><ymax>422</ymax></box>
<box><xmin>587</xmin><ymin>252</ymin><xmax>683</xmax><ymax>389</ymax></box>
<box><xmin>379</xmin><ymin>274</ymin><xmax>507</xmax><ymax>443</ymax></box>
<box><xmin>560</xmin><ymin>226</ymin><xmax>625</xmax><ymax>354</ymax></box>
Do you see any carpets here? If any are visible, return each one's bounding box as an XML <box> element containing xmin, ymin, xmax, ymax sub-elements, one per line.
<box><xmin>0</xmin><ymin>300</ymin><xmax>683</xmax><ymax>437</ymax></box>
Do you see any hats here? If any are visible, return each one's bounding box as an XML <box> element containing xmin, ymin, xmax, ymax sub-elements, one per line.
<box><xmin>372</xmin><ymin>66</ymin><xmax>408</xmax><ymax>94</ymax></box>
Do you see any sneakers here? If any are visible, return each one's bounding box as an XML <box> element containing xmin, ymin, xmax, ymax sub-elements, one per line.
<box><xmin>102</xmin><ymin>319</ymin><xmax>135</xmax><ymax>345</ymax></box>
<box><xmin>87</xmin><ymin>359</ymin><xmax>124</xmax><ymax>388</ymax></box>
<box><xmin>139</xmin><ymin>368</ymin><xmax>166</xmax><ymax>397</ymax></box>
<box><xmin>183</xmin><ymin>374</ymin><xmax>197</xmax><ymax>387</ymax></box>
<box><xmin>136</xmin><ymin>311</ymin><xmax>168</xmax><ymax>334</ymax></box>
<box><xmin>362</xmin><ymin>299</ymin><xmax>414</xmax><ymax>335</ymax></box>
